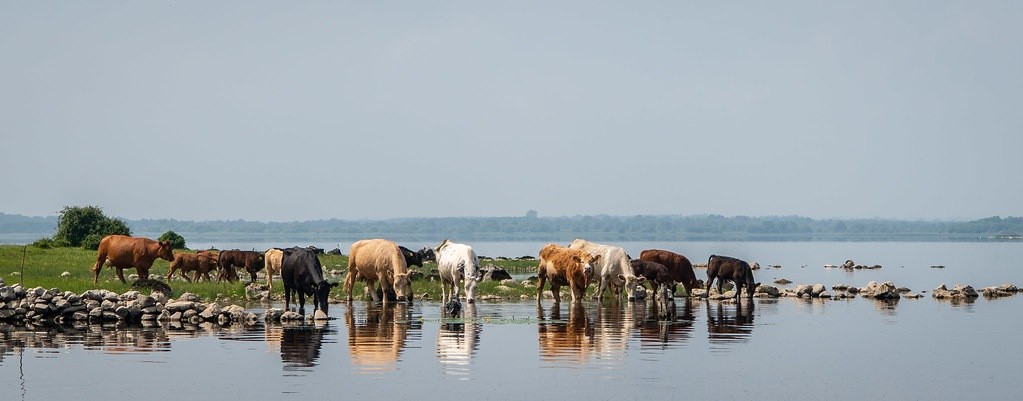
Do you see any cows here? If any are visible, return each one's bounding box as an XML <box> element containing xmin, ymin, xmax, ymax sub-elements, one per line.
<box><xmin>343</xmin><ymin>238</ymin><xmax>423</xmax><ymax>301</ymax></box>
<box><xmin>535</xmin><ymin>238</ymin><xmax>704</xmax><ymax>303</ymax></box>
<box><xmin>166</xmin><ymin>245</ymin><xmax>339</xmax><ymax>308</ymax></box>
<box><xmin>91</xmin><ymin>234</ymin><xmax>176</xmax><ymax>284</ymax></box>
<box><xmin>433</xmin><ymin>239</ymin><xmax>482</xmax><ymax>308</ymax></box>
<box><xmin>704</xmin><ymin>254</ymin><xmax>762</xmax><ymax>298</ymax></box>
